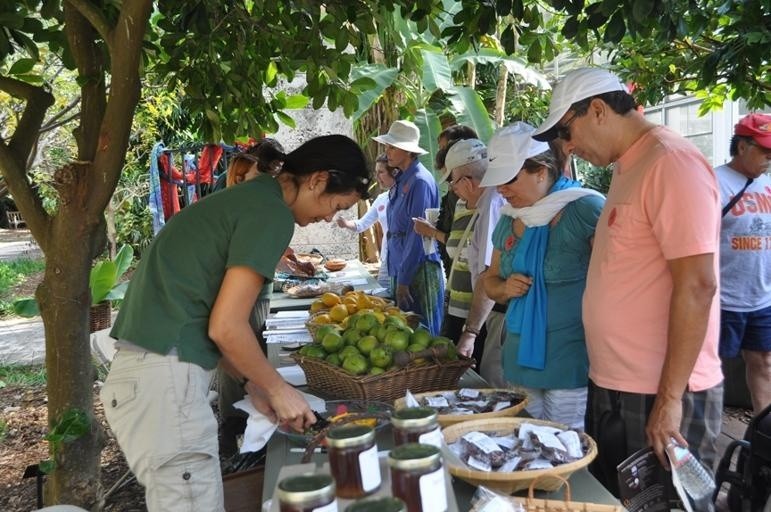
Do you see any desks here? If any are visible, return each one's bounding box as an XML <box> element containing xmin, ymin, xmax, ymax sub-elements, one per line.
<box><xmin>261</xmin><ymin>259</ymin><xmax>627</xmax><ymax>511</ymax></box>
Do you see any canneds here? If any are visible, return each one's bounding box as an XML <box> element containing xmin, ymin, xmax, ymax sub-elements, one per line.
<box><xmin>275</xmin><ymin>406</ymin><xmax>453</xmax><ymax>511</ymax></box>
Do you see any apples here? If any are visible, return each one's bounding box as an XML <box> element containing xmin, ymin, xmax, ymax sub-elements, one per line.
<box><xmin>298</xmin><ymin>310</ymin><xmax>458</xmax><ymax>379</ymax></box>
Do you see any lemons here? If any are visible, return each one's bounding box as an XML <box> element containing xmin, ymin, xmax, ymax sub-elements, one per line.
<box><xmin>307</xmin><ymin>290</ymin><xmax>410</xmax><ymax>328</ymax></box>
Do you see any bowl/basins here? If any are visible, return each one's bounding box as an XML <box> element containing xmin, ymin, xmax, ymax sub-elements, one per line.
<box><xmin>324</xmin><ymin>260</ymin><xmax>350</xmax><ymax>272</ymax></box>
<box><xmin>277</xmin><ymin>398</ymin><xmax>396</xmax><ymax>446</ymax></box>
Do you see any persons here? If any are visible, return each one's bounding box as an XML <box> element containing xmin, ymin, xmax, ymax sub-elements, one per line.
<box><xmin>212</xmin><ymin>138</ymin><xmax>287</xmax><ymax>478</ymax></box>
<box><xmin>532</xmin><ymin>69</ymin><xmax>725</xmax><ymax>512</ymax></box>
<box><xmin>712</xmin><ymin>113</ymin><xmax>771</xmax><ymax>415</ymax></box>
<box><xmin>337</xmin><ymin>120</ymin><xmax>606</xmax><ymax>448</ymax></box>
<box><xmin>101</xmin><ymin>134</ymin><xmax>371</xmax><ymax>512</ymax></box>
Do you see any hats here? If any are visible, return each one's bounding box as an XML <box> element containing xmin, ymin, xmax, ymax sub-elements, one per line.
<box><xmin>477</xmin><ymin>120</ymin><xmax>550</xmax><ymax>188</ymax></box>
<box><xmin>531</xmin><ymin>67</ymin><xmax>631</xmax><ymax>142</ymax></box>
<box><xmin>438</xmin><ymin>139</ymin><xmax>487</xmax><ymax>185</ymax></box>
<box><xmin>734</xmin><ymin>114</ymin><xmax>771</xmax><ymax>149</ymax></box>
<box><xmin>371</xmin><ymin>120</ymin><xmax>430</xmax><ymax>157</ymax></box>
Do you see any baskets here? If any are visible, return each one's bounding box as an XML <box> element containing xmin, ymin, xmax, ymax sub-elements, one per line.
<box><xmin>325</xmin><ymin>261</ymin><xmax>347</xmax><ymax>271</ymax></box>
<box><xmin>278</xmin><ymin>271</ymin><xmax>328</xmax><ymax>285</ymax></box>
<box><xmin>469</xmin><ymin>474</ymin><xmax>621</xmax><ymax>512</ymax></box>
<box><xmin>309</xmin><ymin>296</ymin><xmax>395</xmax><ymax>317</ymax></box>
<box><xmin>87</xmin><ymin>300</ymin><xmax>111</xmax><ymax>333</ymax></box>
<box><xmin>438</xmin><ymin>416</ymin><xmax>598</xmax><ymax>494</ymax></box>
<box><xmin>288</xmin><ymin>348</ymin><xmax>476</xmax><ymax>409</ymax></box>
<box><xmin>393</xmin><ymin>388</ymin><xmax>530</xmax><ymax>428</ymax></box>
<box><xmin>282</xmin><ymin>253</ymin><xmax>323</xmax><ymax>274</ymax></box>
<box><xmin>306</xmin><ymin>311</ymin><xmax>425</xmax><ymax>343</ymax></box>
<box><xmin>282</xmin><ymin>283</ymin><xmax>331</xmax><ymax>298</ymax></box>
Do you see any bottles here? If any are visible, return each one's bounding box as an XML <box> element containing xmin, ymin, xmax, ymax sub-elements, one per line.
<box><xmin>668</xmin><ymin>434</ymin><xmax>718</xmax><ymax>501</ymax></box>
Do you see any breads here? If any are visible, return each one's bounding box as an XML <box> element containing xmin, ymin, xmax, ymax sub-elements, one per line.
<box><xmin>324</xmin><ymin>257</ymin><xmax>346</xmax><ymax>271</ymax></box>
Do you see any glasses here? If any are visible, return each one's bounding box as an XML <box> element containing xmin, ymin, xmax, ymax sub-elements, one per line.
<box><xmin>448</xmin><ymin>176</ymin><xmax>472</xmax><ymax>192</ymax></box>
<box><xmin>553</xmin><ymin>110</ymin><xmax>580</xmax><ymax>141</ymax></box>
<box><xmin>326</xmin><ymin>169</ymin><xmax>370</xmax><ymax>196</ymax></box>
<box><xmin>504</xmin><ymin>160</ymin><xmax>553</xmax><ymax>185</ymax></box>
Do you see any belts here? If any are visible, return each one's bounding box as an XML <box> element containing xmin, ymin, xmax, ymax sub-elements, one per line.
<box><xmin>491</xmin><ymin>304</ymin><xmax>506</xmax><ymax>314</ymax></box>
